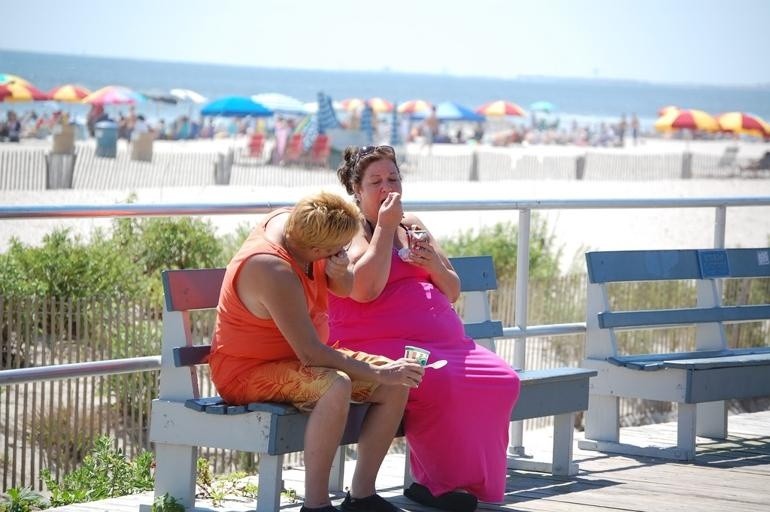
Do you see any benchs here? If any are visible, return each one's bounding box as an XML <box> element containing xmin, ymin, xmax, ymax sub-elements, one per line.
<box><xmin>576</xmin><ymin>246</ymin><xmax>770</xmax><ymax>461</ymax></box>
<box><xmin>138</xmin><ymin>256</ymin><xmax>598</xmax><ymax>512</ymax></box>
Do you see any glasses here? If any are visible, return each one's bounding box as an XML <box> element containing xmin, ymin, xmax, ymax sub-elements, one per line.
<box><xmin>332</xmin><ymin>240</ymin><xmax>352</xmax><ymax>252</ymax></box>
<box><xmin>352</xmin><ymin>145</ymin><xmax>396</xmax><ymax>169</ymax></box>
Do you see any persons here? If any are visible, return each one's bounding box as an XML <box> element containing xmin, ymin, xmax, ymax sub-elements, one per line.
<box><xmin>207</xmin><ymin>191</ymin><xmax>426</xmax><ymax>512</ymax></box>
<box><xmin>530</xmin><ymin>110</ymin><xmax>640</xmax><ymax>143</ymax></box>
<box><xmin>306</xmin><ymin>131</ymin><xmax>330</xmax><ymax>166</ymax></box>
<box><xmin>326</xmin><ymin>144</ymin><xmax>521</xmax><ymax>512</ymax></box>
<box><xmin>1</xmin><ymin>101</ymin><xmax>215</xmax><ymax>141</ymax></box>
<box><xmin>426</xmin><ymin>105</ymin><xmax>439</xmax><ymax>140</ymax></box>
<box><xmin>282</xmin><ymin>135</ymin><xmax>303</xmax><ymax>166</ymax></box>
<box><xmin>227</xmin><ymin>118</ymin><xmax>298</xmax><ymax>138</ymax></box>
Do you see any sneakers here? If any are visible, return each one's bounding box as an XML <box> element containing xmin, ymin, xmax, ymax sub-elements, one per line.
<box><xmin>434</xmin><ymin>493</ymin><xmax>476</xmax><ymax>511</ymax></box>
<box><xmin>300</xmin><ymin>505</ymin><xmax>342</xmax><ymax>512</ymax></box>
<box><xmin>341</xmin><ymin>491</ymin><xmax>405</xmax><ymax>512</ymax></box>
<box><xmin>405</xmin><ymin>483</ymin><xmax>434</xmax><ymax>505</ymax></box>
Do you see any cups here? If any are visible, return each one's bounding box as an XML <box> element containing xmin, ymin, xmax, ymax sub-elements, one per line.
<box><xmin>400</xmin><ymin>345</ymin><xmax>431</xmax><ymax>389</ymax></box>
<box><xmin>405</xmin><ymin>230</ymin><xmax>429</xmax><ymax>258</ymax></box>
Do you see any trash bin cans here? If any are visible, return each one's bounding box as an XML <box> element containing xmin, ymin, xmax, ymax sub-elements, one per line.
<box><xmin>95</xmin><ymin>120</ymin><xmax>117</xmax><ymax>158</ymax></box>
